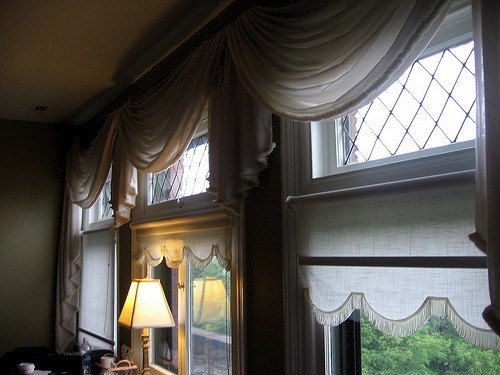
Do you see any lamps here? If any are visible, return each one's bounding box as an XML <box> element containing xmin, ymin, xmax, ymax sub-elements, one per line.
<box><xmin>115</xmin><ymin>278</ymin><xmax>176</xmax><ymax>374</ymax></box>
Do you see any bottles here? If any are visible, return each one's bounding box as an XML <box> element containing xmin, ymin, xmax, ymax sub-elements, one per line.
<box><xmin>79</xmin><ymin>338</ymin><xmax>92</xmax><ymax>374</ymax></box>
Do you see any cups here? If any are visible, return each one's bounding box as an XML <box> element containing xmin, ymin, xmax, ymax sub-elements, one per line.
<box><xmin>19</xmin><ymin>363</ymin><xmax>35</xmax><ymax>375</ymax></box>
<box><xmin>100</xmin><ymin>356</ymin><xmax>116</xmax><ymax>374</ymax></box>
